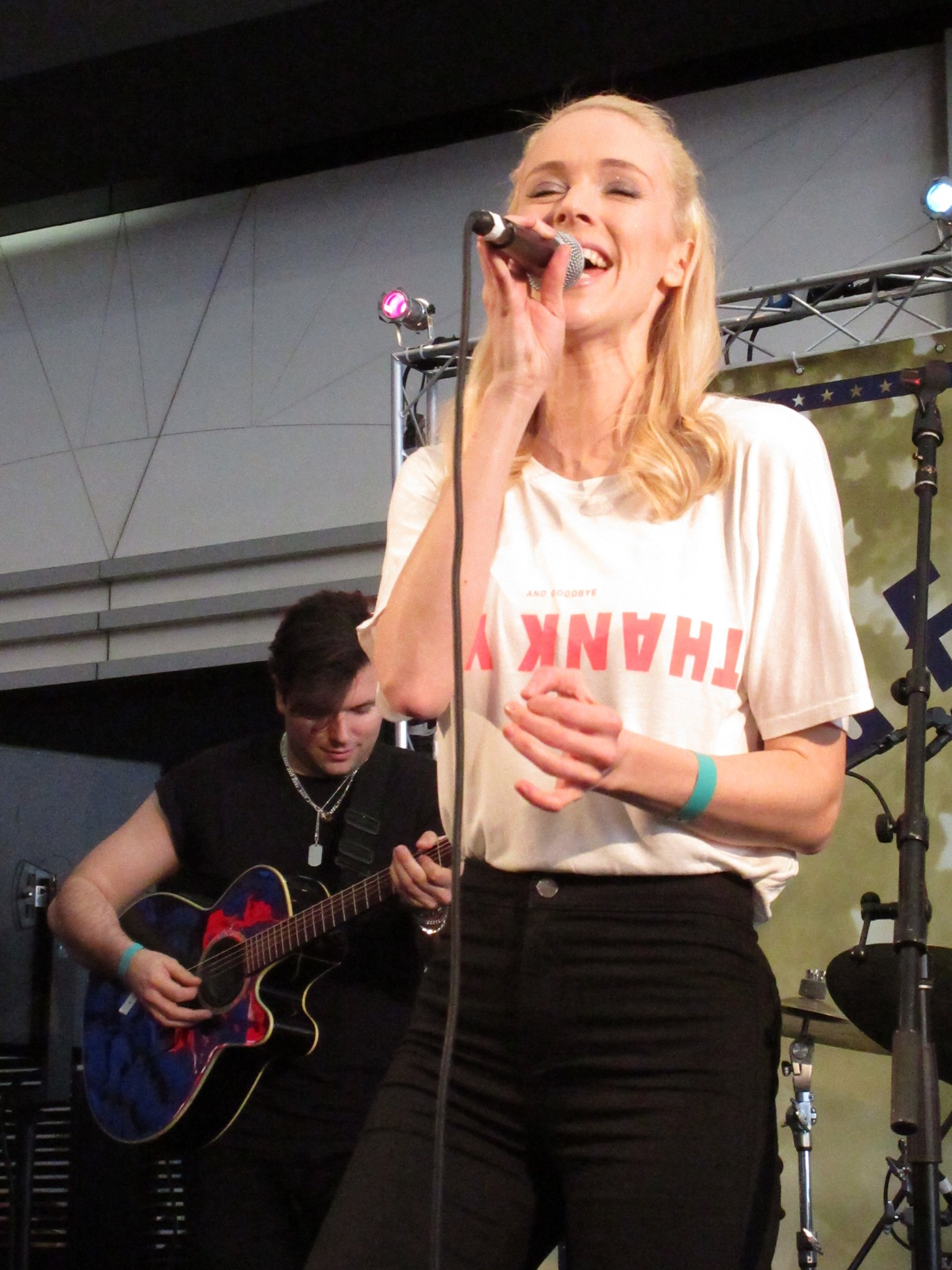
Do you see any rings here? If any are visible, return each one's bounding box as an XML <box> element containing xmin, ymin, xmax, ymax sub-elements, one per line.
<box><xmin>406</xmin><ymin>893</ymin><xmax>416</xmax><ymax>904</ymax></box>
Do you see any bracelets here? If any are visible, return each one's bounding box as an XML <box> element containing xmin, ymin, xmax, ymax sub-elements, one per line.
<box><xmin>414</xmin><ymin>904</ymin><xmax>449</xmax><ymax>937</ymax></box>
<box><xmin>665</xmin><ymin>751</ymin><xmax>718</xmax><ymax>828</ymax></box>
<box><xmin>117</xmin><ymin>943</ymin><xmax>145</xmax><ymax>986</ymax></box>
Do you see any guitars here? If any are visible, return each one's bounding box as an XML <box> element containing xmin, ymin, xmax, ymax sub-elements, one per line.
<box><xmin>85</xmin><ymin>835</ymin><xmax>459</xmax><ymax>1154</ymax></box>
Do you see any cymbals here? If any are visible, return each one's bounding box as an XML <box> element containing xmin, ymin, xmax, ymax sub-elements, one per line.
<box><xmin>779</xmin><ymin>999</ymin><xmax>893</xmax><ymax>1058</ymax></box>
<box><xmin>824</xmin><ymin>941</ymin><xmax>952</xmax><ymax>1085</ymax></box>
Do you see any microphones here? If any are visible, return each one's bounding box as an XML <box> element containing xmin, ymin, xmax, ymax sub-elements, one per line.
<box><xmin>469</xmin><ymin>211</ymin><xmax>586</xmax><ymax>291</ymax></box>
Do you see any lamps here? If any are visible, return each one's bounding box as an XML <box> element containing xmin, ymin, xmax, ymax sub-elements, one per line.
<box><xmin>378</xmin><ymin>287</ymin><xmax>436</xmax><ymax>349</ymax></box>
<box><xmin>920</xmin><ymin>175</ymin><xmax>952</xmax><ymax>251</ymax></box>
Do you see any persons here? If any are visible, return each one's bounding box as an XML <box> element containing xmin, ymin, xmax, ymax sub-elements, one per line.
<box><xmin>303</xmin><ymin>91</ymin><xmax>875</xmax><ymax>1270</ymax></box>
<box><xmin>49</xmin><ymin>588</ymin><xmax>464</xmax><ymax>1270</ymax></box>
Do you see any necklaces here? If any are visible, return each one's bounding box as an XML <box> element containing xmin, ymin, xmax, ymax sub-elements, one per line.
<box><xmin>282</xmin><ymin>731</ymin><xmax>360</xmax><ymax>865</ymax></box>
